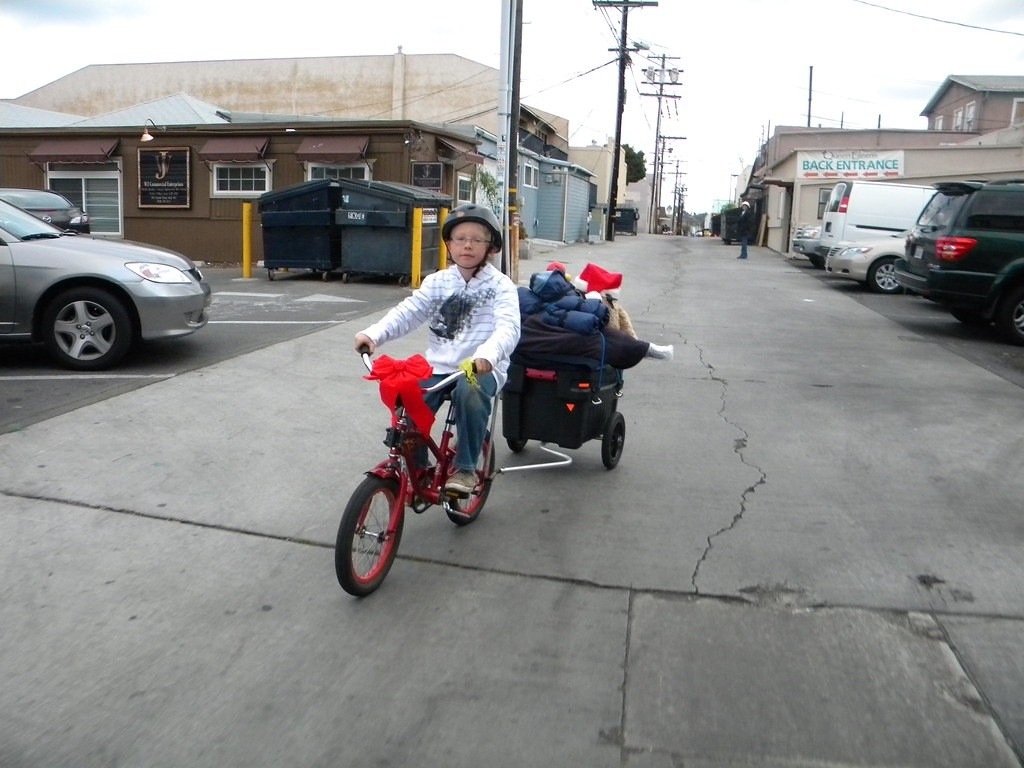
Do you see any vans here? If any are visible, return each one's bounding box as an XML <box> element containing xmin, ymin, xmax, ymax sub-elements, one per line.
<box><xmin>814</xmin><ymin>180</ymin><xmax>941</xmax><ymax>259</ymax></box>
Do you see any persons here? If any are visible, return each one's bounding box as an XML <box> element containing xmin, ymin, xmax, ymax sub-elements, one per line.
<box><xmin>354</xmin><ymin>204</ymin><xmax>520</xmax><ymax>492</ymax></box>
<box><xmin>737</xmin><ymin>201</ymin><xmax>753</xmax><ymax>259</ymax></box>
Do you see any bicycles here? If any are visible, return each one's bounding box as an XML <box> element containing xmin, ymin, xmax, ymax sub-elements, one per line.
<box><xmin>334</xmin><ymin>345</ymin><xmax>511</xmax><ymax>598</ymax></box>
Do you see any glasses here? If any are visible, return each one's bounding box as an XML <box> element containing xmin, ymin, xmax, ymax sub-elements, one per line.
<box><xmin>450</xmin><ymin>236</ymin><xmax>491</xmax><ymax>245</ymax></box>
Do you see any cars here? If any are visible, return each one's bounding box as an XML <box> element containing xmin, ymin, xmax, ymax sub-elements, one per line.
<box><xmin>0</xmin><ymin>197</ymin><xmax>213</xmax><ymax>372</ymax></box>
<box><xmin>661</xmin><ymin>229</ymin><xmax>713</xmax><ymax>238</ymax></box>
<box><xmin>791</xmin><ymin>225</ymin><xmax>827</xmax><ymax>269</ymax></box>
<box><xmin>0</xmin><ymin>186</ymin><xmax>91</xmax><ymax>235</ymax></box>
<box><xmin>822</xmin><ymin>229</ymin><xmax>911</xmax><ymax>297</ymax></box>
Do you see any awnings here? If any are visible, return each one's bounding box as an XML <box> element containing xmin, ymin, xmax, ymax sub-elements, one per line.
<box><xmin>26</xmin><ymin>136</ymin><xmax>121</xmax><ymax>172</ymax></box>
<box><xmin>198</xmin><ymin>136</ymin><xmax>272</xmax><ymax>172</ymax></box>
<box><xmin>294</xmin><ymin>135</ymin><xmax>372</xmax><ymax>172</ymax></box>
<box><xmin>435</xmin><ymin>135</ymin><xmax>484</xmax><ymax>171</ymax></box>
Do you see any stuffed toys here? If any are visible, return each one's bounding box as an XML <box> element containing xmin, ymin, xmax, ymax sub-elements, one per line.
<box><xmin>546</xmin><ymin>261</ymin><xmax>674</xmax><ymax>361</ymax></box>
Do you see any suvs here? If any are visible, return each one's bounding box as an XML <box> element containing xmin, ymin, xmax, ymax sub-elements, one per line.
<box><xmin>894</xmin><ymin>177</ymin><xmax>1024</xmax><ymax>347</ymax></box>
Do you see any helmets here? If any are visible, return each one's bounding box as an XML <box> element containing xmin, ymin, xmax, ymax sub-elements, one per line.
<box><xmin>442</xmin><ymin>204</ymin><xmax>502</xmax><ymax>254</ymax></box>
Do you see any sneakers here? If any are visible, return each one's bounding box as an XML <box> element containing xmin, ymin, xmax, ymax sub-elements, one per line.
<box><xmin>404</xmin><ymin>460</ymin><xmax>432</xmax><ymax>506</ymax></box>
<box><xmin>445</xmin><ymin>467</ymin><xmax>480</xmax><ymax>493</ymax></box>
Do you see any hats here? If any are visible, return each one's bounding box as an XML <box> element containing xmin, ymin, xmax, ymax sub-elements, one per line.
<box><xmin>742</xmin><ymin>201</ymin><xmax>750</xmax><ymax>208</ymax></box>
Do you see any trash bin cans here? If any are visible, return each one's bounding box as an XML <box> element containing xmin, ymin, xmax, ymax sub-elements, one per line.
<box><xmin>710</xmin><ymin>215</ymin><xmax>721</xmax><ymax>237</ymax></box>
<box><xmin>719</xmin><ymin>207</ymin><xmax>744</xmax><ymax>245</ymax></box>
<box><xmin>614</xmin><ymin>208</ymin><xmax>640</xmax><ymax>235</ymax></box>
<box><xmin>257</xmin><ymin>177</ymin><xmax>454</xmax><ymax>287</ymax></box>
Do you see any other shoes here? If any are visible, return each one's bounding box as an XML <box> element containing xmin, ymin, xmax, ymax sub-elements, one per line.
<box><xmin>737</xmin><ymin>256</ymin><xmax>747</xmax><ymax>259</ymax></box>
